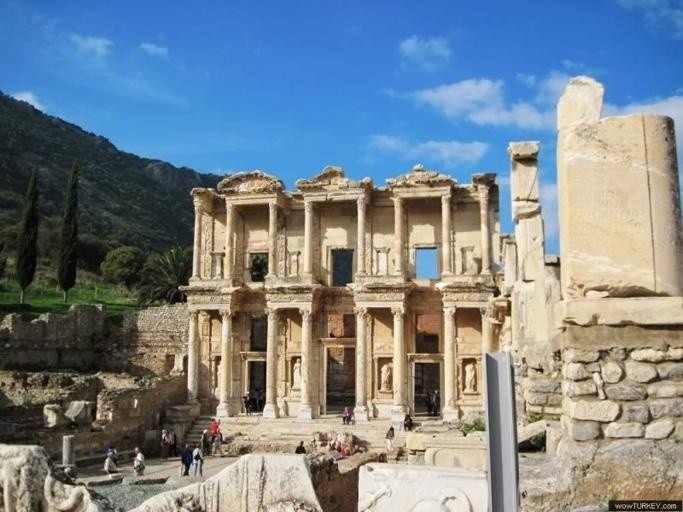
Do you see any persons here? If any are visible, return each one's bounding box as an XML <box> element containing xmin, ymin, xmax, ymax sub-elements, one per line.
<box><xmin>244</xmin><ymin>387</ymin><xmax>264</xmax><ymax>416</ymax></box>
<box><xmin>465</xmin><ymin>363</ymin><xmax>476</xmax><ymax>389</ymax></box>
<box><xmin>426</xmin><ymin>392</ymin><xmax>433</xmax><ymax>416</ymax></box>
<box><xmin>433</xmin><ymin>390</ymin><xmax>439</xmax><ymax>416</ymax></box>
<box><xmin>295</xmin><ymin>441</ymin><xmax>305</xmax><ymax>454</ymax></box>
<box><xmin>342</xmin><ymin>406</ymin><xmax>351</xmax><ymax>424</ymax></box>
<box><xmin>381</xmin><ymin>365</ymin><xmax>391</xmax><ymax>390</ymax></box>
<box><xmin>404</xmin><ymin>414</ymin><xmax>413</xmax><ymax>432</ymax></box>
<box><xmin>160</xmin><ymin>419</ymin><xmax>222</xmax><ymax>478</ymax></box>
<box><xmin>292</xmin><ymin>359</ymin><xmax>301</xmax><ymax>386</ymax></box>
<box><xmin>385</xmin><ymin>427</ymin><xmax>395</xmax><ymax>451</ymax></box>
<box><xmin>133</xmin><ymin>447</ymin><xmax>145</xmax><ymax>475</ymax></box>
<box><xmin>104</xmin><ymin>443</ymin><xmax>118</xmax><ymax>474</ymax></box>
